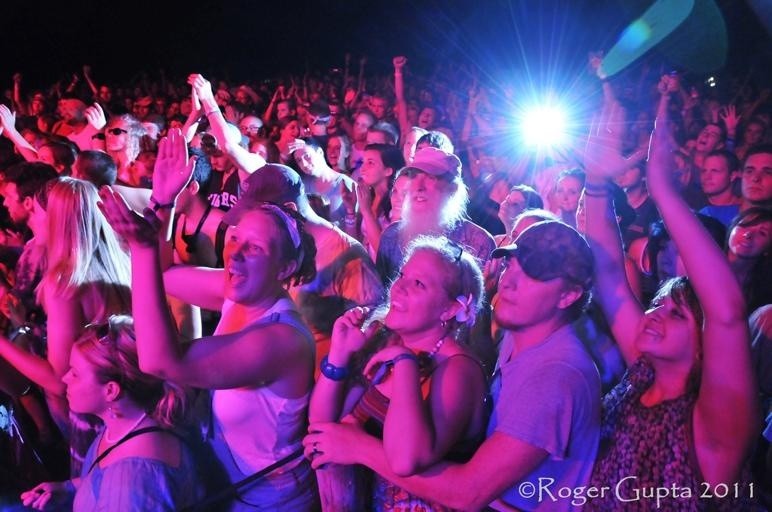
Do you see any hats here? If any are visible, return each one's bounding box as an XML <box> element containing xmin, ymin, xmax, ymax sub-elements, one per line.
<box><xmin>221</xmin><ymin>164</ymin><xmax>305</xmax><ymax>227</ymax></box>
<box><xmin>490</xmin><ymin>220</ymin><xmax>594</xmax><ymax>286</ymax></box>
<box><xmin>400</xmin><ymin>147</ymin><xmax>462</xmax><ymax>178</ymax></box>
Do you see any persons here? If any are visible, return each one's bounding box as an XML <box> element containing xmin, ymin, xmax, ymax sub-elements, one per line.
<box><xmin>0</xmin><ymin>48</ymin><xmax>772</xmax><ymax>511</ymax></box>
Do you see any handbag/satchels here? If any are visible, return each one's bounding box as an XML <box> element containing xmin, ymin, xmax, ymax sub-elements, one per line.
<box><xmin>192</xmin><ymin>437</ymin><xmax>242</xmax><ymax>512</ymax></box>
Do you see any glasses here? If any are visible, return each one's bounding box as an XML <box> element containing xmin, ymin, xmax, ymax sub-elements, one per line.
<box><xmin>108</xmin><ymin>128</ymin><xmax>127</xmax><ymax>135</ymax></box>
<box><xmin>96</xmin><ymin>320</ymin><xmax>124</xmax><ymax>385</ymax></box>
<box><xmin>445</xmin><ymin>242</ymin><xmax>464</xmax><ymax>294</ymax></box>
